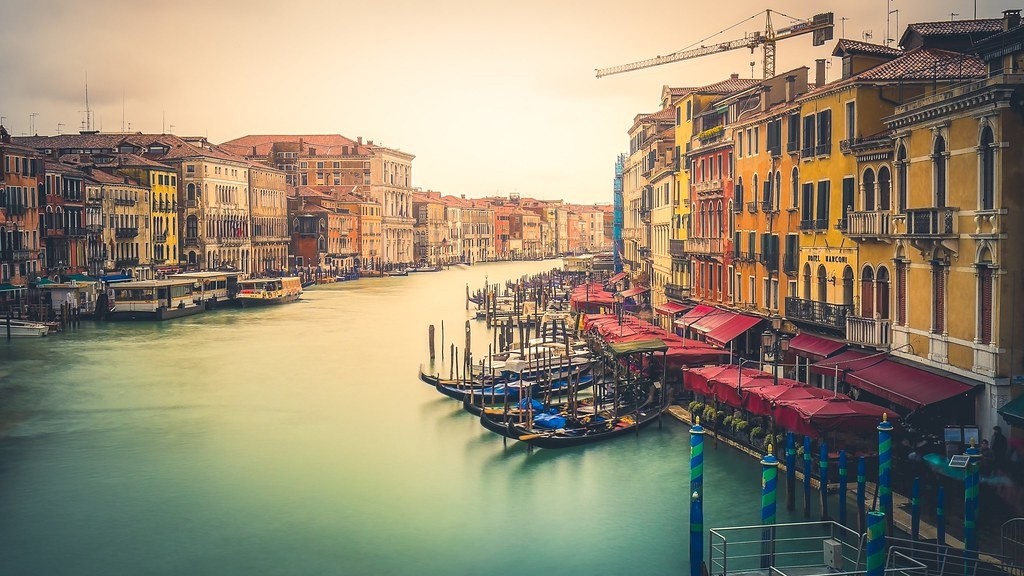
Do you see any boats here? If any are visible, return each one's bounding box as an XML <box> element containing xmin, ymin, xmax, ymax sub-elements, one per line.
<box><xmin>301</xmin><ymin>273</ymin><xmax>359</xmax><ymax>288</ymax></box>
<box><xmin>418</xmin><ymin>253</ymin><xmax>675</xmax><ymax>449</ymax></box>
<box><xmin>360</xmin><ymin>265</ymin><xmax>439</xmax><ymax>277</ymax></box>
<box><xmin>36</xmin><ymin>269</ymin><xmax>304</xmax><ymax>322</ymax></box>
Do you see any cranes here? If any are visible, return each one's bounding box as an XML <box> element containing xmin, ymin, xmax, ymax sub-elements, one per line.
<box><xmin>592</xmin><ymin>5</ymin><xmax>836</xmax><ymax>80</ymax></box>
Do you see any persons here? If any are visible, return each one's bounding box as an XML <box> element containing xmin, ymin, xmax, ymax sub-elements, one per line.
<box><xmin>564</xmin><ymin>291</ymin><xmax>569</xmax><ymax>300</ymax></box>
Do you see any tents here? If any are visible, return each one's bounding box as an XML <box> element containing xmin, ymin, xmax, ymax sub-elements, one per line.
<box><xmin>570</xmin><ymin>281</ymin><xmax>620</xmax><ymax>315</ymax></box>
<box><xmin>582</xmin><ymin>311</ymin><xmax>901</xmax><ymax>446</ymax></box>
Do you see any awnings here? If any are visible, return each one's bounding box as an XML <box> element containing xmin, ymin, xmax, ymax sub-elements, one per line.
<box><xmin>655</xmin><ymin>301</ymin><xmax>695</xmax><ymax>317</ymax></box>
<box><xmin>690</xmin><ymin>309</ymin><xmax>737</xmax><ymax>335</ymax></box>
<box><xmin>704</xmin><ymin>315</ymin><xmax>763</xmax><ymax>349</ymax></box>
<box><xmin>101</xmin><ymin>275</ymin><xmax>132</xmax><ymax>282</ymax></box>
<box><xmin>619</xmin><ymin>285</ymin><xmax>649</xmax><ymax>300</ymax></box>
<box><xmin>844</xmin><ymin>360</ymin><xmax>976</xmax><ymax>414</ymax></box>
<box><xmin>608</xmin><ymin>272</ymin><xmax>629</xmax><ymax>286</ymax></box>
<box><xmin>785</xmin><ymin>331</ymin><xmax>847</xmax><ymax>361</ymax></box>
<box><xmin>673</xmin><ymin>303</ymin><xmax>718</xmax><ymax>330</ymax></box>
<box><xmin>0</xmin><ymin>283</ymin><xmax>28</xmax><ymax>290</ymax></box>
<box><xmin>64</xmin><ymin>274</ymin><xmax>98</xmax><ymax>283</ymax></box>
<box><xmin>811</xmin><ymin>350</ymin><xmax>887</xmax><ymax>380</ymax></box>
<box><xmin>30</xmin><ymin>278</ymin><xmax>57</xmax><ymax>284</ymax></box>
